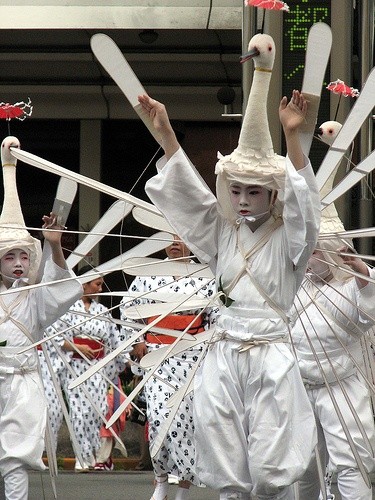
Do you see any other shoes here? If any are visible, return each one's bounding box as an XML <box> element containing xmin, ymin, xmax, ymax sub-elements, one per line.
<box><xmin>104</xmin><ymin>456</ymin><xmax>114</xmax><ymax>471</ymax></box>
<box><xmin>94</xmin><ymin>463</ymin><xmax>104</xmax><ymax>469</ymax></box>
<box><xmin>74</xmin><ymin>465</ymin><xmax>89</xmax><ymax>472</ymax></box>
<box><xmin>151</xmin><ymin>495</ymin><xmax>167</xmax><ymax>500</ymax></box>
<box><xmin>88</xmin><ymin>465</ymin><xmax>94</xmax><ymax>470</ymax></box>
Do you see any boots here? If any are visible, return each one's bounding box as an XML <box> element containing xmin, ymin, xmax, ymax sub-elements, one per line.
<box><xmin>135</xmin><ymin>442</ymin><xmax>153</xmax><ymax>470</ymax></box>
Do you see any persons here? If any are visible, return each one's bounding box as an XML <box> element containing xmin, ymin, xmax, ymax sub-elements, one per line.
<box><xmin>119</xmin><ymin>234</ymin><xmax>220</xmax><ymax>500</ymax></box>
<box><xmin>94</xmin><ymin>376</ymin><xmax>132</xmax><ymax>472</ymax></box>
<box><xmin>0</xmin><ymin>213</ymin><xmax>84</xmax><ymax>500</ymax></box>
<box><xmin>289</xmin><ymin>243</ymin><xmax>375</xmax><ymax>500</ymax></box>
<box><xmin>43</xmin><ymin>265</ymin><xmax>134</xmax><ymax>472</ymax></box>
<box><xmin>138</xmin><ymin>90</ymin><xmax>321</xmax><ymax>500</ymax></box>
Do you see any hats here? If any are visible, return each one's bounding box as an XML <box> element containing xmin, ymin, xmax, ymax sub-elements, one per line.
<box><xmin>0</xmin><ymin>136</ymin><xmax>34</xmax><ymax>258</ymax></box>
<box><xmin>314</xmin><ymin>121</ymin><xmax>353</xmax><ymax>251</ymax></box>
<box><xmin>215</xmin><ymin>33</ymin><xmax>286</xmax><ymax>189</ymax></box>
